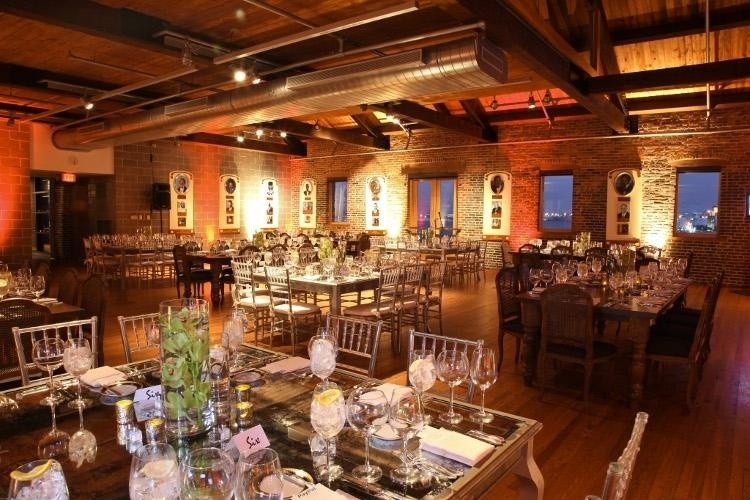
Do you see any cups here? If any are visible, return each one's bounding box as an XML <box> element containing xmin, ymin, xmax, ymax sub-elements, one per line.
<box><xmin>409</xmin><ymin>349</ymin><xmax>436</xmax><ymax>426</ymax></box>
<box><xmin>265</xmin><ymin>252</ymin><xmax>272</xmax><ymax>267</ymax></box>
<box><xmin>253</xmin><ymin>252</ymin><xmax>262</xmax><ymax>272</ymax></box>
<box><xmin>436</xmin><ymin>350</ymin><xmax>469</xmax><ymax>424</ymax></box>
<box><xmin>63</xmin><ymin>338</ymin><xmax>93</xmax><ymax>409</ymax></box>
<box><xmin>386</xmin><ymin>387</ymin><xmax>424</xmax><ymax>484</ymax></box>
<box><xmin>129</xmin><ymin>443</ymin><xmax>180</xmax><ymax>500</ymax></box>
<box><xmin>68</xmin><ymin>404</ymin><xmax>98</xmax><ymax>469</ymax></box>
<box><xmin>32</xmin><ymin>339</ymin><xmax>65</xmax><ymax>405</ymax></box>
<box><xmin>529</xmin><ymin>268</ymin><xmax>541</xmax><ymax>298</ymax></box>
<box><xmin>91</xmin><ymin>233</ymin><xmax>195</xmax><ymax>246</ymax></box>
<box><xmin>346</xmin><ymin>388</ymin><xmax>389</xmax><ymax>483</ymax></box>
<box><xmin>236</xmin><ymin>448</ymin><xmax>285</xmax><ymax>500</ymax></box>
<box><xmin>7</xmin><ymin>459</ymin><xmax>71</xmax><ymax>500</ymax></box>
<box><xmin>221</xmin><ymin>309</ymin><xmax>247</xmax><ymax>370</ymax></box>
<box><xmin>176</xmin><ymin>447</ymin><xmax>237</xmax><ymax>500</ymax></box>
<box><xmin>539</xmin><ymin>269</ymin><xmax>554</xmax><ymax>288</ymax></box>
<box><xmin>209</xmin><ymin>333</ymin><xmax>230</xmax><ymax>399</ymax></box>
<box><xmin>315</xmin><ymin>255</ymin><xmax>373</xmax><ymax>281</ymax></box>
<box><xmin>309</xmin><ymin>431</ymin><xmax>337</xmax><ymax>473</ymax></box>
<box><xmin>29</xmin><ymin>276</ymin><xmax>46</xmax><ymax>304</ymax></box>
<box><xmin>376</xmin><ymin>252</ymin><xmax>420</xmax><ymax>270</ymax></box>
<box><xmin>469</xmin><ymin>348</ymin><xmax>497</xmax><ymax>424</ymax></box>
<box><xmin>308</xmin><ymin>326</ymin><xmax>338</xmax><ymax>382</ymax></box>
<box><xmin>0</xmin><ymin>264</ymin><xmax>32</xmax><ymax>300</ymax></box>
<box><xmin>37</xmin><ymin>407</ymin><xmax>70</xmax><ymax>467</ymax></box>
<box><xmin>272</xmin><ymin>248</ymin><xmax>319</xmax><ymax>266</ymax></box>
<box><xmin>309</xmin><ymin>383</ymin><xmax>345</xmax><ymax>480</ymax></box>
<box><xmin>528</xmin><ymin>239</ymin><xmax>625</xmax><ymax>255</ymax></box>
<box><xmin>551</xmin><ymin>257</ymin><xmax>689</xmax><ymax>301</ymax></box>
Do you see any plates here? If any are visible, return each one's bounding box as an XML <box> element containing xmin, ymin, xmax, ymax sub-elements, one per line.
<box><xmin>252</xmin><ymin>467</ymin><xmax>313</xmax><ymax>499</ymax></box>
<box><xmin>231</xmin><ymin>368</ymin><xmax>268</xmax><ymax>386</ymax></box>
<box><xmin>98</xmin><ymin>380</ymin><xmax>142</xmax><ymax>398</ymax></box>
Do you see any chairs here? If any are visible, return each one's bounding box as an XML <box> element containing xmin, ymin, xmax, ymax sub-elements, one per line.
<box><xmin>266</xmin><ymin>244</ymin><xmax>288</xmax><ymax>266</ymax></box>
<box><xmin>518</xmin><ymin>243</ymin><xmax>542</xmax><ymax>291</ymax></box>
<box><xmin>418</xmin><ymin>261</ymin><xmax>444</xmax><ymax>338</ymax></box>
<box><xmin>80</xmin><ymin>273</ymin><xmax>109</xmax><ymax>367</ymax></box>
<box><xmin>537</xmin><ymin>282</ymin><xmax>617</xmax><ymax>402</ymax></box>
<box><xmin>343</xmin><ymin>266</ymin><xmax>401</xmax><ymax>353</ymax></box>
<box><xmin>181</xmin><ymin>240</ymin><xmax>315</xmax><ymax>251</ymax></box>
<box><xmin>584</xmin><ymin>246</ymin><xmax>608</xmax><ymax>261</ymax></box>
<box><xmin>660</xmin><ymin>271</ymin><xmax>724</xmax><ymax>364</ymax></box>
<box><xmin>230</xmin><ymin>260</ymin><xmax>288</xmax><ymax>347</ymax></box>
<box><xmin>231</xmin><ymin>255</ymin><xmax>259</xmax><ymax>272</ymax></box>
<box><xmin>684</xmin><ymin>252</ymin><xmax>694</xmax><ymax>277</ymax></box>
<box><xmin>440</xmin><ymin>234</ymin><xmax>487</xmax><ymax>289</ymax></box>
<box><xmin>314</xmin><ymin>232</ymin><xmax>371</xmax><ymax>255</ymax></box>
<box><xmin>267</xmin><ymin>253</ymin><xmax>292</xmax><ymax>267</ymax></box>
<box><xmin>637</xmin><ymin>246</ymin><xmax>662</xmax><ymax>259</ymax></box>
<box><xmin>519</xmin><ymin>253</ymin><xmax>541</xmax><ymax>269</ymax></box>
<box><xmin>265</xmin><ymin>229</ymin><xmax>308</xmax><ymax>238</ymax></box>
<box><xmin>382</xmin><ymin>263</ymin><xmax>424</xmax><ymax>349</ymax></box>
<box><xmin>493</xmin><ymin>267</ymin><xmax>525</xmax><ymax>370</ymax></box>
<box><xmin>585</xmin><ymin>412</ymin><xmax>651</xmax><ymax>500</ymax></box>
<box><xmin>81</xmin><ymin>231</ymin><xmax>176</xmax><ymax>286</ymax></box>
<box><xmin>644</xmin><ymin>278</ymin><xmax>719</xmax><ymax>411</ymax></box>
<box><xmin>35</xmin><ymin>264</ymin><xmax>52</xmax><ymax>296</ymax></box>
<box><xmin>0</xmin><ymin>298</ymin><xmax>52</xmax><ymax>388</ymax></box>
<box><xmin>12</xmin><ymin>315</ymin><xmax>99</xmax><ymax>386</ymax></box>
<box><xmin>244</xmin><ymin>260</ymin><xmax>418</xmax><ymax>342</ymax></box>
<box><xmin>174</xmin><ymin>234</ymin><xmax>203</xmax><ymax>251</ymax></box>
<box><xmin>501</xmin><ymin>240</ymin><xmax>513</xmax><ymax>267</ymax></box>
<box><xmin>296</xmin><ymin>250</ymin><xmax>322</xmax><ymax>264</ymax></box>
<box><xmin>407</xmin><ymin>329</ymin><xmax>485</xmax><ymax>404</ymax></box>
<box><xmin>117</xmin><ymin>312</ymin><xmax>164</xmax><ymax>364</ymax></box>
<box><xmin>221</xmin><ymin>245</ymin><xmax>261</xmax><ymax>305</ymax></box>
<box><xmin>317</xmin><ymin>312</ymin><xmax>384</xmax><ymax>378</ymax></box>
<box><xmin>378</xmin><ymin>233</ymin><xmax>443</xmax><ymax>263</ymax></box>
<box><xmin>261</xmin><ymin>265</ymin><xmax>323</xmax><ymax>355</ymax></box>
<box><xmin>171</xmin><ymin>245</ymin><xmax>211</xmax><ymax>302</ymax></box>
<box><xmin>56</xmin><ymin>268</ymin><xmax>81</xmax><ymax>308</ymax></box>
<box><xmin>634</xmin><ymin>259</ymin><xmax>660</xmax><ymax>273</ymax></box>
<box><xmin>553</xmin><ymin>244</ymin><xmax>575</xmax><ymax>279</ymax></box>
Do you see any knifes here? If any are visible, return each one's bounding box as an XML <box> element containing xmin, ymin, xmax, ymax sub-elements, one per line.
<box><xmin>95</xmin><ymin>382</ymin><xmax>122</xmax><ymax>396</ymax></box>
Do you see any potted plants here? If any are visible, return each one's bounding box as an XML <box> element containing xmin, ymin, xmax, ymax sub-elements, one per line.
<box><xmin>157</xmin><ymin>304</ymin><xmax>209</xmax><ymax>433</ymax></box>
